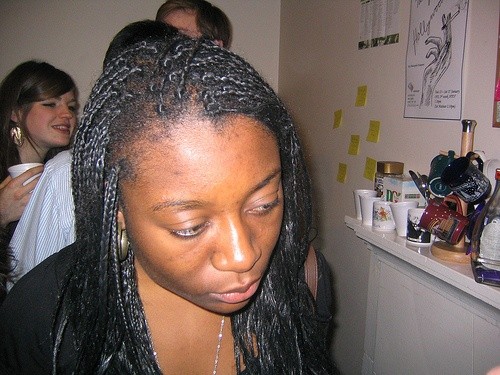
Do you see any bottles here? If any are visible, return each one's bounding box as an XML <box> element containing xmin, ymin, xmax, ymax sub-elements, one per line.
<box><xmin>470</xmin><ymin>168</ymin><xmax>500</xmax><ymax>287</ymax></box>
<box><xmin>374</xmin><ymin>161</ymin><xmax>405</xmax><ymax>198</ymax></box>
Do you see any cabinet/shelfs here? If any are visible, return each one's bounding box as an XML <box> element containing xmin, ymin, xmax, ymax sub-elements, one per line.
<box><xmin>340</xmin><ymin>216</ymin><xmax>500</xmax><ymax>375</ymax></box>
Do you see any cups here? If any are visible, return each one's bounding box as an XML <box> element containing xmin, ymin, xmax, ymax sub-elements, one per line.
<box><xmin>7</xmin><ymin>163</ymin><xmax>43</xmax><ymax>193</ymax></box>
<box><xmin>406</xmin><ymin>208</ymin><xmax>441</xmax><ymax>247</ymax></box>
<box><xmin>353</xmin><ymin>189</ymin><xmax>377</xmax><ymax>221</ymax></box>
<box><xmin>359</xmin><ymin>195</ymin><xmax>381</xmax><ymax>227</ymax></box>
<box><xmin>419</xmin><ymin>195</ymin><xmax>471</xmax><ymax>245</ymax></box>
<box><xmin>427</xmin><ymin>150</ymin><xmax>456</xmax><ymax>198</ymax></box>
<box><xmin>390</xmin><ymin>201</ymin><xmax>419</xmax><ymax>237</ymax></box>
<box><xmin>441</xmin><ymin>151</ymin><xmax>492</xmax><ymax>205</ymax></box>
<box><xmin>372</xmin><ymin>201</ymin><xmax>395</xmax><ymax>231</ymax></box>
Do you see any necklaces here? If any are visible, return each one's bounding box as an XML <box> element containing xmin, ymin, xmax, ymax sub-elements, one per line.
<box><xmin>142</xmin><ymin>306</ymin><xmax>225</xmax><ymax>375</ymax></box>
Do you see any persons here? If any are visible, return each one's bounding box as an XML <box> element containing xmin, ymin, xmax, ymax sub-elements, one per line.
<box><xmin>0</xmin><ymin>0</ymin><xmax>337</xmax><ymax>375</ymax></box>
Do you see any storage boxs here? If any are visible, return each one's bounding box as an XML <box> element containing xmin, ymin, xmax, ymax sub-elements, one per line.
<box><xmin>372</xmin><ymin>172</ymin><xmax>426</xmax><ymax>209</ymax></box>
